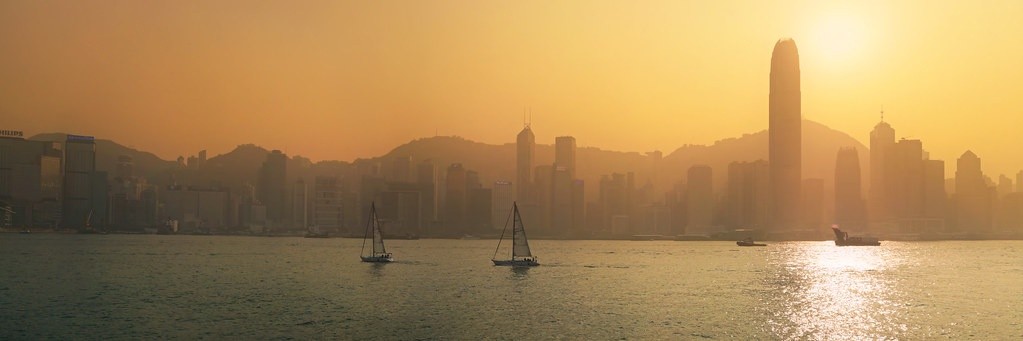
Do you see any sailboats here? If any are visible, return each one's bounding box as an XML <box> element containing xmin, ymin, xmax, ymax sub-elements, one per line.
<box><xmin>359</xmin><ymin>201</ymin><xmax>395</xmax><ymax>263</ymax></box>
<box><xmin>491</xmin><ymin>200</ymin><xmax>538</xmax><ymax>266</ymax></box>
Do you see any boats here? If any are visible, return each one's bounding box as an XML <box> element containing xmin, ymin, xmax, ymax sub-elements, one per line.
<box><xmin>832</xmin><ymin>225</ymin><xmax>881</xmax><ymax>246</ymax></box>
<box><xmin>737</xmin><ymin>237</ymin><xmax>766</xmax><ymax>246</ymax></box>
